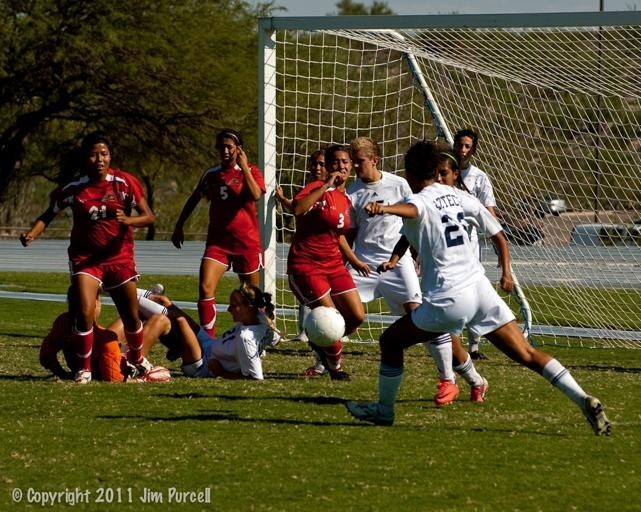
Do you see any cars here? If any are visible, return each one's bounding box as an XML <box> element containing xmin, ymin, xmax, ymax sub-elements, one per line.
<box><xmin>512</xmin><ymin>195</ymin><xmax>566</xmax><ymax>219</ymax></box>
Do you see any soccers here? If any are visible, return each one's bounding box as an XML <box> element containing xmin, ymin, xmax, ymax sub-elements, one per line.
<box><xmin>305</xmin><ymin>306</ymin><xmax>346</xmax><ymax>347</ymax></box>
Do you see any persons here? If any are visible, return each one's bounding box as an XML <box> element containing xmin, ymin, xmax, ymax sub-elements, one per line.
<box><xmin>347</xmin><ymin>140</ymin><xmax>610</xmax><ymax>439</ymax></box>
<box><xmin>172</xmin><ymin>129</ymin><xmax>282</xmax><ymax>349</ymax></box>
<box><xmin>296</xmin><ymin>137</ymin><xmax>413</xmax><ymax>375</ymax></box>
<box><xmin>376</xmin><ymin>149</ymin><xmax>514</xmax><ymax>409</ymax></box>
<box><xmin>436</xmin><ymin>130</ymin><xmax>498</xmax><ymax>363</ymax></box>
<box><xmin>286</xmin><ymin>142</ymin><xmax>371</xmax><ymax>381</ymax></box>
<box><xmin>19</xmin><ymin>134</ymin><xmax>155</xmax><ymax>384</ymax></box>
<box><xmin>137</xmin><ymin>285</ymin><xmax>280</xmax><ymax>380</ymax></box>
<box><xmin>39</xmin><ymin>281</ymin><xmax>182</xmax><ymax>385</ymax></box>
<box><xmin>277</xmin><ymin>146</ymin><xmax>328</xmax><ymax>342</ymax></box>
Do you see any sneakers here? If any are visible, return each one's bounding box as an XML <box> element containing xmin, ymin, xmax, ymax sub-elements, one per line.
<box><xmin>330</xmin><ymin>370</ymin><xmax>351</xmax><ymax>382</ymax></box>
<box><xmin>582</xmin><ymin>395</ymin><xmax>611</xmax><ymax>436</ymax></box>
<box><xmin>434</xmin><ymin>382</ymin><xmax>459</xmax><ymax>407</ymax></box>
<box><xmin>345</xmin><ymin>400</ymin><xmax>394</xmax><ymax>427</ymax></box>
<box><xmin>470</xmin><ymin>377</ymin><xmax>488</xmax><ymax>402</ymax></box>
<box><xmin>130</xmin><ymin>355</ymin><xmax>154</xmax><ymax>375</ymax></box>
<box><xmin>301</xmin><ymin>366</ymin><xmax>323</xmax><ymax>377</ymax></box>
<box><xmin>468</xmin><ymin>350</ymin><xmax>488</xmax><ymax>361</ymax></box>
<box><xmin>151</xmin><ymin>283</ymin><xmax>164</xmax><ymax>294</ymax></box>
<box><xmin>295</xmin><ymin>331</ymin><xmax>309</xmax><ymax>343</ymax></box>
<box><xmin>74</xmin><ymin>369</ymin><xmax>92</xmax><ymax>385</ymax></box>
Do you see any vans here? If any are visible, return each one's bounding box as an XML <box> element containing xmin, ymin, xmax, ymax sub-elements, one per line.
<box><xmin>570</xmin><ymin>223</ymin><xmax>641</xmax><ymax>246</ymax></box>
